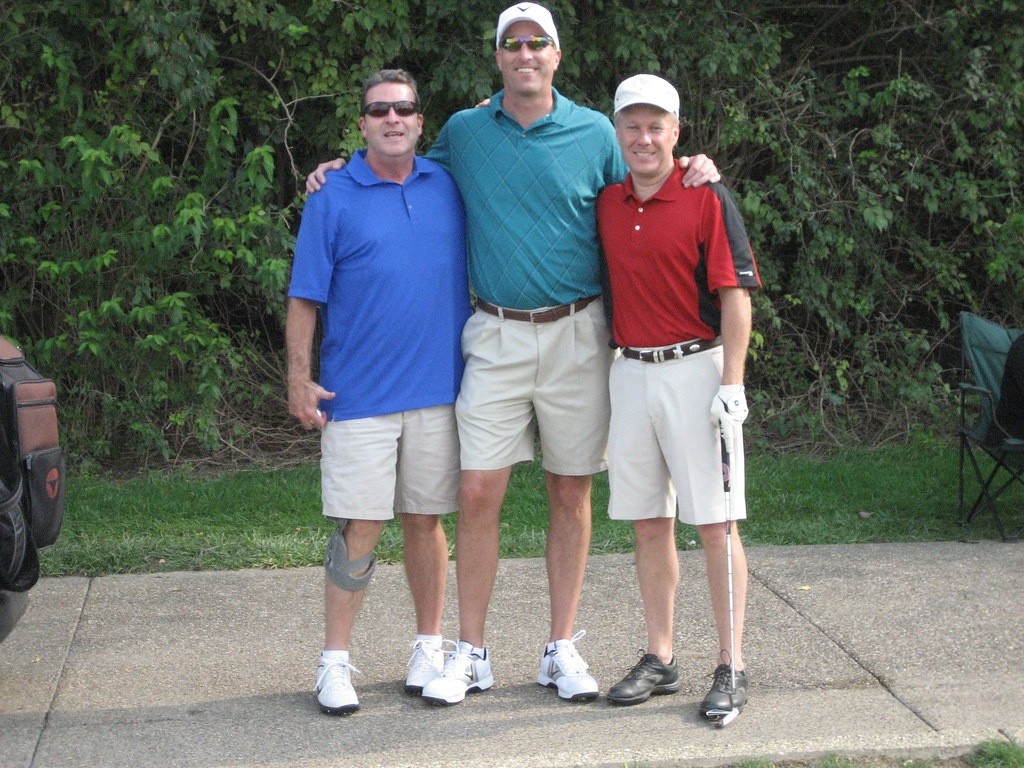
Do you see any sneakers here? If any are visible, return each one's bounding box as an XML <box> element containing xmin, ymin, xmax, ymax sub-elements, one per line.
<box><xmin>313</xmin><ymin>656</ymin><xmax>362</xmax><ymax>714</ymax></box>
<box><xmin>536</xmin><ymin>630</ymin><xmax>599</xmax><ymax>702</ymax></box>
<box><xmin>404</xmin><ymin>639</ymin><xmax>444</xmax><ymax>695</ymax></box>
<box><xmin>699</xmin><ymin>648</ymin><xmax>748</xmax><ymax>718</ymax></box>
<box><xmin>422</xmin><ymin>638</ymin><xmax>494</xmax><ymax>705</ymax></box>
<box><xmin>606</xmin><ymin>648</ymin><xmax>679</xmax><ymax>705</ymax></box>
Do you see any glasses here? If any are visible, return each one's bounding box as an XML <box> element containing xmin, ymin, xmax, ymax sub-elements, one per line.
<box><xmin>499</xmin><ymin>34</ymin><xmax>555</xmax><ymax>51</ymax></box>
<box><xmin>360</xmin><ymin>101</ymin><xmax>421</xmax><ymax>118</ymax></box>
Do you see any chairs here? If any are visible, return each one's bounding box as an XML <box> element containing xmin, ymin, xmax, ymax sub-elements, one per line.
<box><xmin>957</xmin><ymin>311</ymin><xmax>1024</xmax><ymax>541</ymax></box>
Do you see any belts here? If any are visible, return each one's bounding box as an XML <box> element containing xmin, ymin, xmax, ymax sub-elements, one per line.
<box><xmin>477</xmin><ymin>294</ymin><xmax>601</xmax><ymax>324</ymax></box>
<box><xmin>608</xmin><ymin>335</ymin><xmax>723</xmax><ymax>363</ymax></box>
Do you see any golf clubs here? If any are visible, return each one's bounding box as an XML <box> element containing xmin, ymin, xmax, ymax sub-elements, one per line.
<box><xmin>714</xmin><ymin>411</ymin><xmax>742</xmax><ymax>730</ymax></box>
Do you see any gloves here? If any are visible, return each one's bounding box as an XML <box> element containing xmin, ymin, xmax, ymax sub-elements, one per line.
<box><xmin>710</xmin><ymin>385</ymin><xmax>749</xmax><ymax>428</ymax></box>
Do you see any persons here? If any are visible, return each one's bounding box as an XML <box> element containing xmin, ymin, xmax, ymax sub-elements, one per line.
<box><xmin>982</xmin><ymin>333</ymin><xmax>1024</xmax><ymax>448</ymax></box>
<box><xmin>305</xmin><ymin>1</ymin><xmax>723</xmax><ymax>706</ymax></box>
<box><xmin>284</xmin><ymin>67</ymin><xmax>474</xmax><ymax>717</ymax></box>
<box><xmin>596</xmin><ymin>73</ymin><xmax>753</xmax><ymax>719</ymax></box>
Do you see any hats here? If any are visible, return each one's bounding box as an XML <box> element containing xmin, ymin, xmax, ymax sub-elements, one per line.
<box><xmin>614</xmin><ymin>73</ymin><xmax>680</xmax><ymax>116</ymax></box>
<box><xmin>495</xmin><ymin>2</ymin><xmax>560</xmax><ymax>51</ymax></box>
